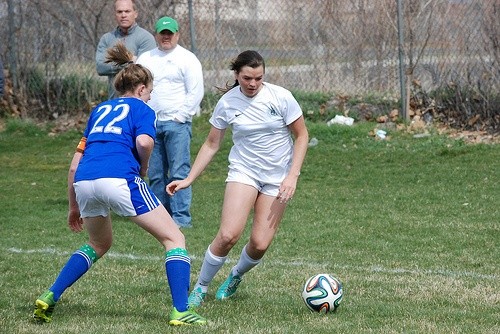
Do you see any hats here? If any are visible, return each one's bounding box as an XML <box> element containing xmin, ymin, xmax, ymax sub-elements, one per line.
<box><xmin>154</xmin><ymin>16</ymin><xmax>179</xmax><ymax>34</ymax></box>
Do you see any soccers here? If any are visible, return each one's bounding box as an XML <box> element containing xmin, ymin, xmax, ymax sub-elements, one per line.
<box><xmin>301</xmin><ymin>273</ymin><xmax>344</xmax><ymax>313</ymax></box>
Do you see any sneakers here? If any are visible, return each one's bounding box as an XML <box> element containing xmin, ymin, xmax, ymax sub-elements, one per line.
<box><xmin>188</xmin><ymin>283</ymin><xmax>209</xmax><ymax>308</ymax></box>
<box><xmin>168</xmin><ymin>302</ymin><xmax>210</xmax><ymax>327</ymax></box>
<box><xmin>32</xmin><ymin>289</ymin><xmax>58</xmax><ymax>325</ymax></box>
<box><xmin>215</xmin><ymin>265</ymin><xmax>245</xmax><ymax>300</ymax></box>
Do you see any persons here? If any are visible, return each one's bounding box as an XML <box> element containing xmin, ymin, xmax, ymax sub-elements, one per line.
<box><xmin>165</xmin><ymin>50</ymin><xmax>310</xmax><ymax>310</ymax></box>
<box><xmin>32</xmin><ymin>40</ymin><xmax>216</xmax><ymax>330</ymax></box>
<box><xmin>94</xmin><ymin>0</ymin><xmax>157</xmax><ymax>102</ymax></box>
<box><xmin>135</xmin><ymin>16</ymin><xmax>206</xmax><ymax>230</ymax></box>
